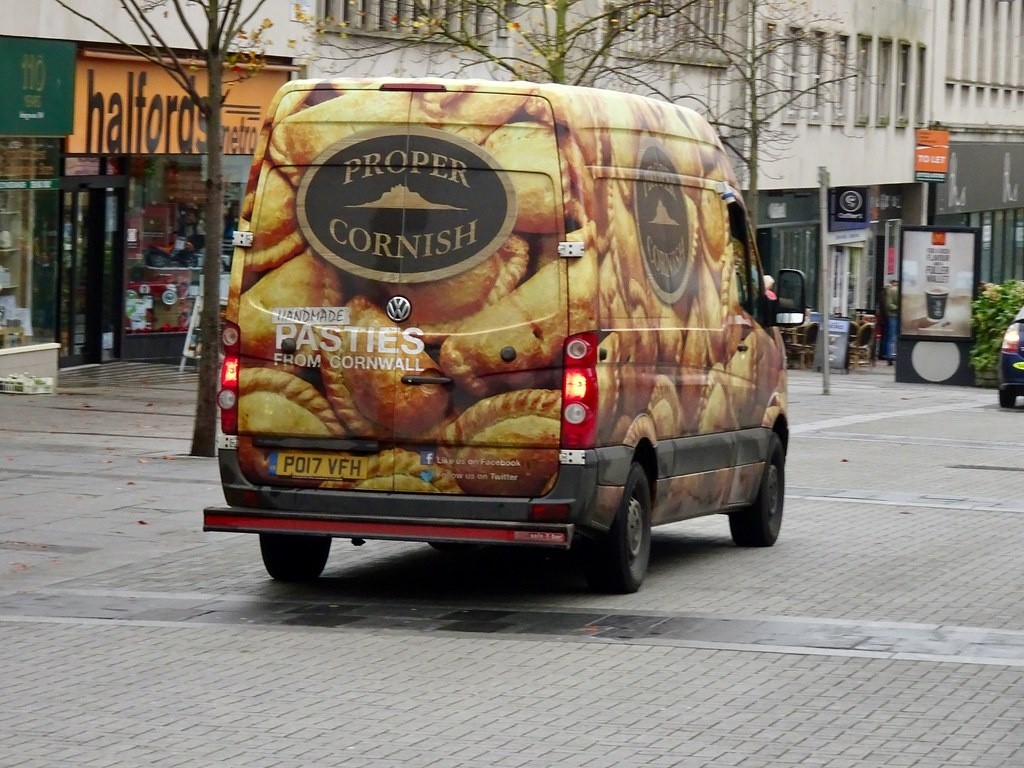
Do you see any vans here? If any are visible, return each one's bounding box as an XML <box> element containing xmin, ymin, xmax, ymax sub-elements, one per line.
<box><xmin>200</xmin><ymin>71</ymin><xmax>809</xmax><ymax>597</ymax></box>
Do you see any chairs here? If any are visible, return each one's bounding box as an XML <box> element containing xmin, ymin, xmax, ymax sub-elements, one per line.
<box><xmin>780</xmin><ymin>321</ymin><xmax>875</xmax><ymax>370</ymax></box>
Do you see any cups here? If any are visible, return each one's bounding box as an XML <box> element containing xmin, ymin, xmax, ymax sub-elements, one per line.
<box><xmin>924</xmin><ymin>286</ymin><xmax>950</xmax><ymax>322</ymax></box>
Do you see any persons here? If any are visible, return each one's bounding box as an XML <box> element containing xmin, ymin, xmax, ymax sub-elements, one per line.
<box><xmin>884</xmin><ymin>280</ymin><xmax>898</xmax><ymax>356</ymax></box>
<box><xmin>222</xmin><ymin>203</ymin><xmax>240</xmax><ymax>272</ymax></box>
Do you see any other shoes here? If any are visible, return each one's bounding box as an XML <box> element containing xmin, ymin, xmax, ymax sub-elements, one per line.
<box><xmin>878</xmin><ymin>356</ymin><xmax>887</xmax><ymax>360</ymax></box>
<box><xmin>887</xmin><ymin>357</ymin><xmax>893</xmax><ymax>366</ymax></box>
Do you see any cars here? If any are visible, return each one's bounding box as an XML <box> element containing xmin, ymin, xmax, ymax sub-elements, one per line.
<box><xmin>996</xmin><ymin>302</ymin><xmax>1024</xmax><ymax>409</ymax></box>
